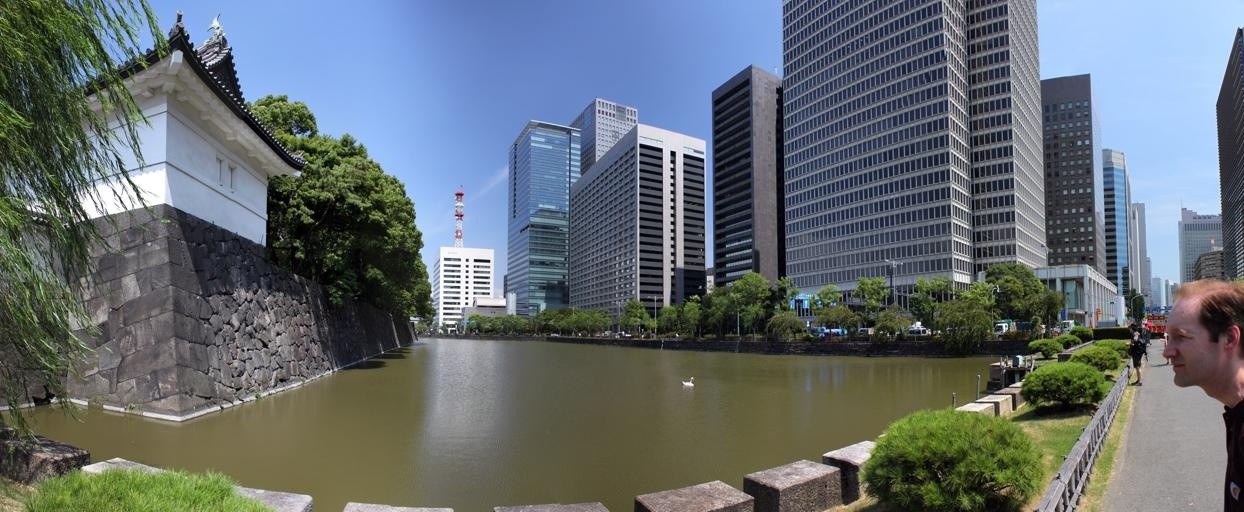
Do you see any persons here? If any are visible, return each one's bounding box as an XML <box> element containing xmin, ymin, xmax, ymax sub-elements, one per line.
<box><xmin>1163</xmin><ymin>332</ymin><xmax>1170</xmax><ymax>365</ymax></box>
<box><xmin>413</xmin><ymin>323</ymin><xmax>1081</xmax><ymax>345</ymax></box>
<box><xmin>1159</xmin><ymin>273</ymin><xmax>1244</xmax><ymax>512</ymax></box>
<box><xmin>1129</xmin><ymin>323</ymin><xmax>1152</xmax><ymax>386</ymax></box>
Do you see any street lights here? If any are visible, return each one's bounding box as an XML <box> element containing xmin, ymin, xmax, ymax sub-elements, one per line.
<box><xmin>648</xmin><ymin>296</ymin><xmax>664</xmax><ymax>334</ymax></box>
<box><xmin>991</xmin><ymin>285</ymin><xmax>1001</xmax><ymax>334</ymax></box>
<box><xmin>1040</xmin><ymin>241</ymin><xmax>1052</xmax><ymax>338</ymax></box>
<box><xmin>611</xmin><ymin>302</ymin><xmax>626</xmax><ymax>331</ymax></box>
<box><xmin>884</xmin><ymin>258</ymin><xmax>904</xmax><ymax>303</ymax></box>
<box><xmin>1131</xmin><ymin>293</ymin><xmax>1149</xmax><ymax>325</ymax></box>
<box><xmin>567</xmin><ymin>306</ymin><xmax>579</xmax><ymax>316</ymax></box>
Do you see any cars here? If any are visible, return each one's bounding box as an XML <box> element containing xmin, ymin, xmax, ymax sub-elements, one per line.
<box><xmin>810</xmin><ymin>319</ymin><xmax>1082</xmax><ymax>339</ymax></box>
<box><xmin>549</xmin><ymin>330</ymin><xmax>633</xmax><ymax>339</ymax></box>
